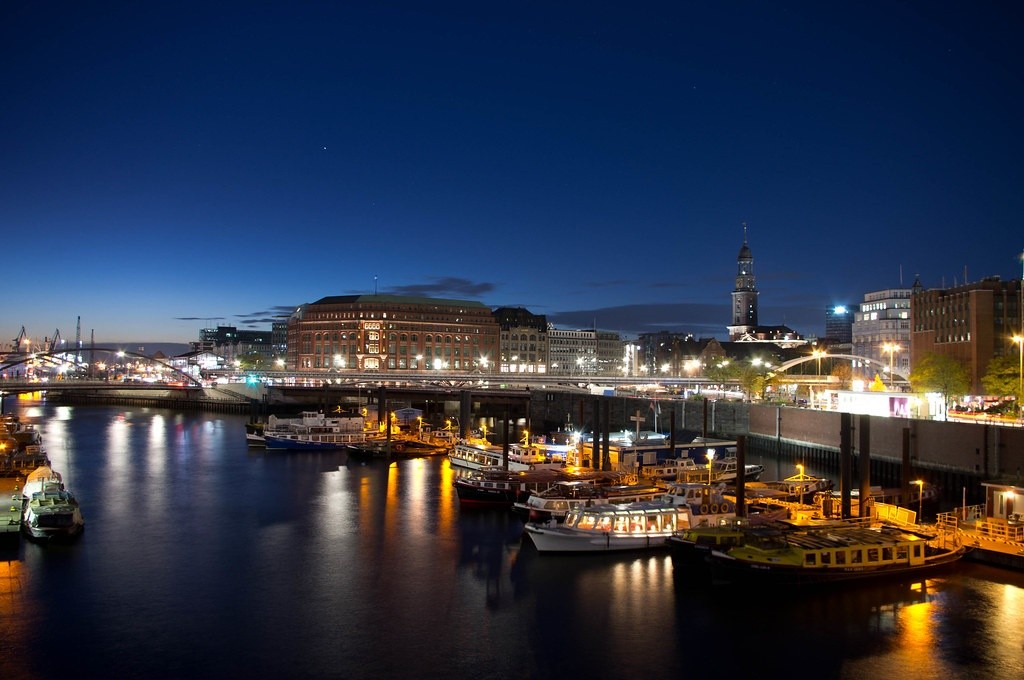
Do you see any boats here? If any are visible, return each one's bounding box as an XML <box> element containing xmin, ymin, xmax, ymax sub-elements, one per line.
<box><xmin>452</xmin><ymin>468</ymin><xmax>527</xmax><ymax>511</ymax></box>
<box><xmin>642</xmin><ymin>456</ymin><xmax>765</xmax><ymax>483</ymax></box>
<box><xmin>245</xmin><ymin>405</ymin><xmax>453</xmax><ymax>457</ymax></box>
<box><xmin>513</xmin><ymin>481</ymin><xmax>670</xmax><ymax>524</ymax></box>
<box><xmin>668</xmin><ymin>523</ymin><xmax>747</xmax><ymax>564</ymax></box>
<box><xmin>21</xmin><ymin>465</ymin><xmax>85</xmax><ymax>546</ymax></box>
<box><xmin>11</xmin><ymin>425</ymin><xmax>51</xmax><ymax>476</ymax></box>
<box><xmin>708</xmin><ymin>522</ymin><xmax>967</xmax><ymax>587</ymax></box>
<box><xmin>522</xmin><ymin>503</ymin><xmax>694</xmax><ymax>553</ymax></box>
<box><xmin>448</xmin><ymin>442</ymin><xmax>567</xmax><ymax>473</ymax></box>
<box><xmin>585</xmin><ymin>483</ymin><xmax>736</xmax><ymax>516</ymax></box>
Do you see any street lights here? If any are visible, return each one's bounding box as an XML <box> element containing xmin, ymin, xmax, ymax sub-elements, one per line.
<box><xmin>523</xmin><ymin>430</ymin><xmax>529</xmax><ymax>445</ymax></box>
<box><xmin>1012</xmin><ymin>337</ymin><xmax>1024</xmax><ymax>392</ymax></box>
<box><xmin>447</xmin><ymin>422</ymin><xmax>451</xmax><ymax>431</ymax></box>
<box><xmin>884</xmin><ymin>343</ymin><xmax>901</xmax><ymax>388</ymax></box>
<box><xmin>482</xmin><ymin>426</ymin><xmax>486</xmax><ymax>439</ymax></box>
<box><xmin>797</xmin><ymin>464</ymin><xmax>805</xmax><ymax>504</ymax></box>
<box><xmin>418</xmin><ymin>417</ymin><xmax>422</xmax><ymax>428</ymax></box>
<box><xmin>916</xmin><ymin>479</ymin><xmax>924</xmax><ymax>524</ymax></box>
<box><xmin>707</xmin><ymin>449</ymin><xmax>716</xmax><ymax>485</ymax></box>
<box><xmin>814</xmin><ymin>351</ymin><xmax>826</xmax><ymax>376</ymax></box>
